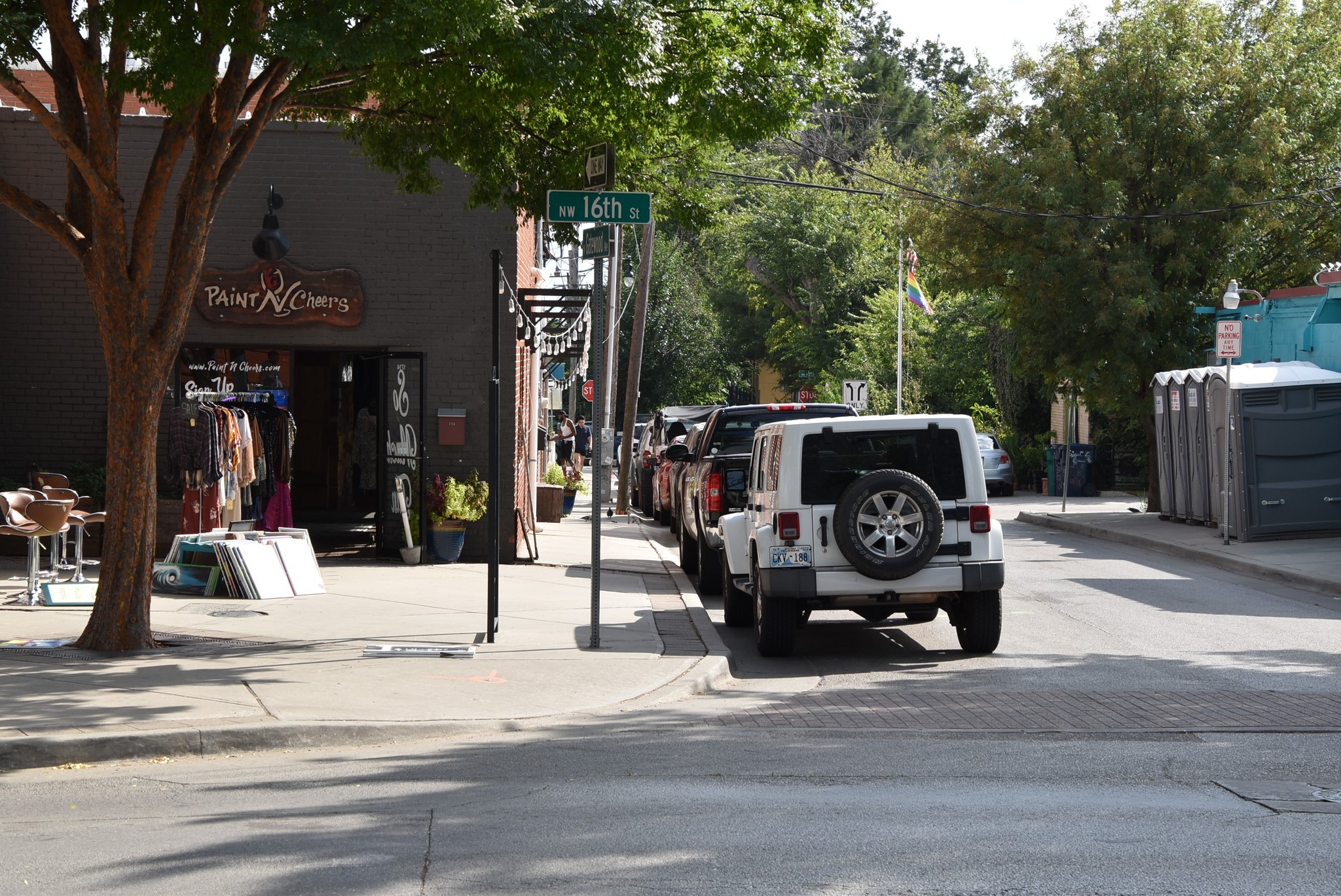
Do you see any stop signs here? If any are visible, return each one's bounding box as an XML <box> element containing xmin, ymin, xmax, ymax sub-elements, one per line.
<box><xmin>799</xmin><ymin>386</ymin><xmax>817</xmax><ymax>403</ymax></box>
<box><xmin>582</xmin><ymin>142</ymin><xmax>615</xmax><ymax>190</ymax></box>
<box><xmin>581</xmin><ymin>379</ymin><xmax>597</xmax><ymax>402</ymax></box>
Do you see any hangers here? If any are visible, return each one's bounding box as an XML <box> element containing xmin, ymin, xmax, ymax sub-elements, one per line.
<box><xmin>358</xmin><ymin>400</ymin><xmax>379</xmax><ymax>417</ymax></box>
<box><xmin>167</xmin><ymin>392</ymin><xmax>294</xmax><ymax>421</ymax></box>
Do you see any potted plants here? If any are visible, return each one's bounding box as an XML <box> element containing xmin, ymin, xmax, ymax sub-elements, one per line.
<box><xmin>426</xmin><ymin>466</ymin><xmax>489</xmax><ymax>564</ymax></box>
<box><xmin>995</xmin><ymin>426</ymin><xmax>1057</xmax><ymax>495</ymax></box>
<box><xmin>541</xmin><ymin>461</ymin><xmax>590</xmax><ymax>517</ymax></box>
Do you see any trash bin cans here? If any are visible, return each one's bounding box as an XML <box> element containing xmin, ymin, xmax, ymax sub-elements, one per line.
<box><xmin>1045</xmin><ymin>445</ymin><xmax>1055</xmax><ymax>496</ymax></box>
<box><xmin>1052</xmin><ymin>443</ymin><xmax>1065</xmax><ymax>497</ymax></box>
<box><xmin>1058</xmin><ymin>444</ymin><xmax>1097</xmax><ymax>497</ymax></box>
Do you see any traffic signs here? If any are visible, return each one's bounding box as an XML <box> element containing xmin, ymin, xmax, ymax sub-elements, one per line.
<box><xmin>583</xmin><ymin>224</ymin><xmax>617</xmax><ymax>260</ymax></box>
<box><xmin>798</xmin><ymin>370</ymin><xmax>816</xmax><ymax>377</ymax></box>
<box><xmin>546</xmin><ymin>189</ymin><xmax>652</xmax><ymax>225</ymax></box>
<box><xmin>1217</xmin><ymin>320</ymin><xmax>1242</xmax><ymax>358</ymax></box>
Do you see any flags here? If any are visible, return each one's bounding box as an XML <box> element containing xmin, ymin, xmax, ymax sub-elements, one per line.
<box><xmin>906</xmin><ymin>235</ymin><xmax>935</xmax><ymax>315</ymax></box>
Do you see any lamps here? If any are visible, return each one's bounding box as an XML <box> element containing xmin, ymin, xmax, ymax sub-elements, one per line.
<box><xmin>1222</xmin><ymin>279</ymin><xmax>1263</xmax><ymax>309</ymax></box>
<box><xmin>252</xmin><ymin>182</ymin><xmax>291</xmax><ymax>263</ymax></box>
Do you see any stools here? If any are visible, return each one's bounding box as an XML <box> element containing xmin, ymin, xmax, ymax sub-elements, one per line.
<box><xmin>0</xmin><ymin>472</ymin><xmax>106</xmax><ymax>606</ymax></box>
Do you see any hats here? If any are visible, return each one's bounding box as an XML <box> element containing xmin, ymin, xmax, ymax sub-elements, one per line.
<box><xmin>558</xmin><ymin>410</ymin><xmax>569</xmax><ymax>417</ymax></box>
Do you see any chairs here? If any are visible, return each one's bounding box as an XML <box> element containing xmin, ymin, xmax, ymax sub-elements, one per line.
<box><xmin>665</xmin><ymin>422</ymin><xmax>688</xmax><ymax>444</ymax></box>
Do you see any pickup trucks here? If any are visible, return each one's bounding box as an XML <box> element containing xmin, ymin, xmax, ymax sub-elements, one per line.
<box><xmin>664</xmin><ymin>401</ymin><xmax>867</xmax><ymax>598</ymax></box>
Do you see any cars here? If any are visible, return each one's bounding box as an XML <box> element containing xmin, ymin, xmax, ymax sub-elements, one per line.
<box><xmin>973</xmin><ymin>433</ymin><xmax>1015</xmax><ymax>497</ymax></box>
<box><xmin>617</xmin><ymin>405</ymin><xmax>809</xmax><ymax>578</ymax></box>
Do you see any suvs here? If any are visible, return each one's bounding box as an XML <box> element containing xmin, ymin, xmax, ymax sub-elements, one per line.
<box><xmin>717</xmin><ymin>414</ymin><xmax>1006</xmax><ymax>660</ymax></box>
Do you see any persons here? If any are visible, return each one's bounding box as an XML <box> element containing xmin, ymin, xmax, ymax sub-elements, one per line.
<box><xmin>574</xmin><ymin>415</ymin><xmax>593</xmax><ymax>475</ymax></box>
<box><xmin>546</xmin><ymin>411</ymin><xmax>579</xmax><ymax>482</ymax></box>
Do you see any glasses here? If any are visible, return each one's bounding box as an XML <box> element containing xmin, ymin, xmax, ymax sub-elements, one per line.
<box><xmin>578</xmin><ymin>421</ymin><xmax>584</xmax><ymax>424</ymax></box>
